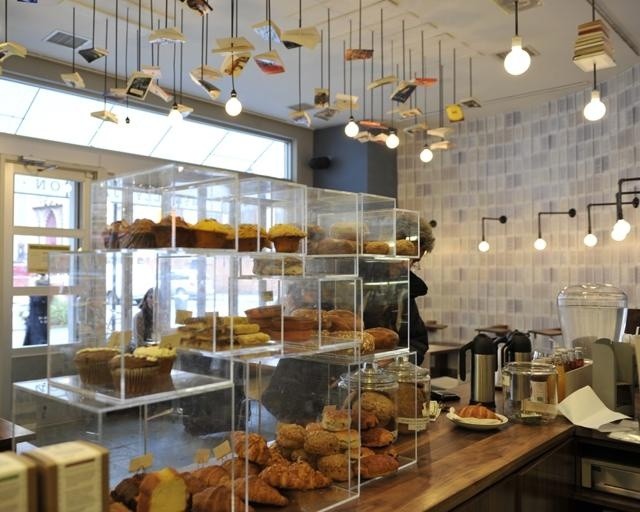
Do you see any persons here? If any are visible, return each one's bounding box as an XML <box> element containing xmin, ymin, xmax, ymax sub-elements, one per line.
<box><xmin>21</xmin><ymin>277</ymin><xmax>54</xmax><ymax>345</ymax></box>
<box><xmin>262</xmin><ymin>209</ymin><xmax>435</xmax><ymax>427</ymax></box>
<box><xmin>132</xmin><ymin>287</ymin><xmax>171</xmax><ymax>348</ymax></box>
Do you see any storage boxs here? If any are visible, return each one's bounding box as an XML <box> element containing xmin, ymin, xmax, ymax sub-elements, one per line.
<box><xmin>552</xmin><ymin>355</ymin><xmax>595</xmax><ymax>406</ymax></box>
<box><xmin>0</xmin><ymin>450</ymin><xmax>43</xmax><ymax>512</ymax></box>
<box><xmin>20</xmin><ymin>438</ymin><xmax>112</xmax><ymax>512</ymax></box>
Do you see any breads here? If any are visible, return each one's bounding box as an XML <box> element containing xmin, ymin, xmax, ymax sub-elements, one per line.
<box><xmin>178</xmin><ymin>316</ymin><xmax>271</xmax><ymax>350</ymax></box>
<box><xmin>290</xmin><ymin>308</ymin><xmax>399</xmax><ymax>355</ymax></box>
<box><xmin>100</xmin><ymin>215</ymin><xmax>306</xmax><ymax>253</ymax></box>
<box><xmin>272</xmin><ymin>410</ymin><xmax>399</xmax><ymax>481</ymax></box>
<box><xmin>75</xmin><ymin>344</ymin><xmax>177</xmax><ymax>395</ymax></box>
<box><xmin>244</xmin><ymin>305</ymin><xmax>314</xmax><ymax>342</ymax></box>
<box><xmin>456</xmin><ymin>404</ymin><xmax>503</xmax><ymax>422</ymax></box>
<box><xmin>304</xmin><ymin>220</ymin><xmax>416</xmax><ymax>257</ymax></box>
<box><xmin>111</xmin><ymin>434</ymin><xmax>333</xmax><ymax>512</ymax></box>
<box><xmin>343</xmin><ymin>389</ymin><xmax>397</xmax><ymax>447</ymax></box>
<box><xmin>384</xmin><ymin>384</ymin><xmax>424</xmax><ymax>433</ymax></box>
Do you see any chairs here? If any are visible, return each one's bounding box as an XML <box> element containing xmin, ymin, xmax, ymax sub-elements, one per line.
<box><xmin>169</xmin><ymin>352</ymin><xmax>252</xmax><ymax>439</ymax></box>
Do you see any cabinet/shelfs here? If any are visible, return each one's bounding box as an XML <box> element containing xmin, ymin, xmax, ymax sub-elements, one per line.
<box><xmin>450</xmin><ymin>432</ymin><xmax>579</xmax><ymax>512</ymax></box>
<box><xmin>21</xmin><ymin>160</ymin><xmax>423</xmax><ymax>511</ymax></box>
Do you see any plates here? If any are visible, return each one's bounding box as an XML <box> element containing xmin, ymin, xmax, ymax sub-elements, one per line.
<box><xmin>446</xmin><ymin>413</ymin><xmax>508</xmax><ymax>429</ymax></box>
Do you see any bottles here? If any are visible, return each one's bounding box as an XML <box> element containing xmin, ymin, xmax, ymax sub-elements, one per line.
<box><xmin>549</xmin><ymin>346</ymin><xmax>584</xmax><ymax>370</ymax></box>
<box><xmin>494</xmin><ymin>329</ymin><xmax>531</xmax><ymax>408</ymax></box>
<box><xmin>530</xmin><ymin>352</ymin><xmax>548</xmax><ymax>409</ymax></box>
<box><xmin>383</xmin><ymin>354</ymin><xmax>433</xmax><ymax>437</ymax></box>
<box><xmin>555</xmin><ymin>281</ymin><xmax>629</xmax><ymax>357</ymax></box>
<box><xmin>459</xmin><ymin>333</ymin><xmax>498</xmax><ymax>407</ymax></box>
<box><xmin>336</xmin><ymin>362</ymin><xmax>400</xmax><ymax>452</ymax></box>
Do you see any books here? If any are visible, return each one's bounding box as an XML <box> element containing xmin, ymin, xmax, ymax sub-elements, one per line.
<box><xmin>572</xmin><ymin>18</ymin><xmax>619</xmax><ymax>74</ymax></box>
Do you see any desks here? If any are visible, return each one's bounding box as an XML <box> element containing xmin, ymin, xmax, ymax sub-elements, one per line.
<box><xmin>474</xmin><ymin>324</ymin><xmax>513</xmax><ymax>343</ymax></box>
<box><xmin>420</xmin><ymin>338</ymin><xmax>468</xmax><ymax>384</ymax></box>
<box><xmin>527</xmin><ymin>327</ymin><xmax>564</xmax><ymax>351</ymax></box>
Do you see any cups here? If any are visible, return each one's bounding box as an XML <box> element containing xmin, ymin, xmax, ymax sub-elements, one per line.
<box><xmin>502</xmin><ymin>361</ymin><xmax>559</xmax><ymax>425</ymax></box>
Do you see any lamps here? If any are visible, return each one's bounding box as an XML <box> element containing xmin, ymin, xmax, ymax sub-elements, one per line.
<box><xmin>162</xmin><ymin>1</ymin><xmax>486</xmax><ymax>163</ymax></box>
<box><xmin>475</xmin><ymin>175</ymin><xmax>640</xmax><ymax>263</ymax></box>
<box><xmin>569</xmin><ymin>1</ymin><xmax>621</xmax><ymax>124</ymax></box>
<box><xmin>501</xmin><ymin>1</ymin><xmax>534</xmax><ymax>78</ymax></box>
